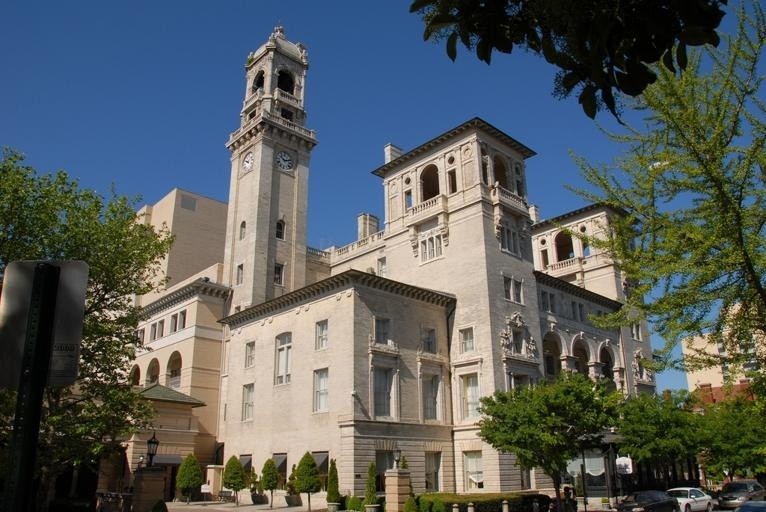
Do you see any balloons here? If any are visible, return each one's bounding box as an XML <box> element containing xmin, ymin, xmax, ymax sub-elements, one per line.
<box><xmin>240</xmin><ymin>151</ymin><xmax>254</xmax><ymax>171</ymax></box>
<box><xmin>274</xmin><ymin>150</ymin><xmax>293</xmax><ymax>171</ymax></box>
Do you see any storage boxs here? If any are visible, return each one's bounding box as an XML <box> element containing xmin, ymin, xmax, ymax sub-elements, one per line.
<box><xmin>215</xmin><ymin>491</ymin><xmax>231</xmax><ymax>503</ymax></box>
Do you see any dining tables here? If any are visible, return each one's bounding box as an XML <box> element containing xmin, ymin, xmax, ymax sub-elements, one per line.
<box><xmin>325</xmin><ymin>458</ymin><xmax>341</xmax><ymax>512</ymax></box>
<box><xmin>601</xmin><ymin>498</ymin><xmax>609</xmax><ymax>510</ymax></box>
<box><xmin>363</xmin><ymin>462</ymin><xmax>381</xmax><ymax>512</ymax></box>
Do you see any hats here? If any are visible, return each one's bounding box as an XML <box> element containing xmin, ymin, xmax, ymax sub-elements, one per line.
<box><xmin>666</xmin><ymin>487</ymin><xmax>715</xmax><ymax>512</ymax></box>
<box><xmin>734</xmin><ymin>501</ymin><xmax>766</xmax><ymax>512</ymax></box>
<box><xmin>715</xmin><ymin>478</ymin><xmax>762</xmax><ymax>508</ymax></box>
<box><xmin>616</xmin><ymin>490</ymin><xmax>680</xmax><ymax>512</ymax></box>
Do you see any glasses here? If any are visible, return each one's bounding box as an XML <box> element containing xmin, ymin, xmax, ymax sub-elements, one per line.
<box><xmin>145</xmin><ymin>431</ymin><xmax>158</xmax><ymax>466</ymax></box>
<box><xmin>392</xmin><ymin>441</ymin><xmax>401</xmax><ymax>468</ymax></box>
